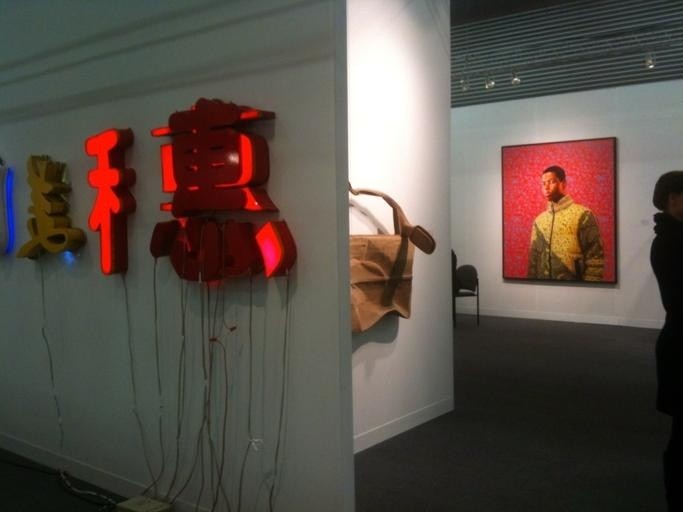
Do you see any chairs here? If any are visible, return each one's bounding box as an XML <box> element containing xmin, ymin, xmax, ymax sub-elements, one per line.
<box><xmin>454</xmin><ymin>263</ymin><xmax>482</xmax><ymax>327</ymax></box>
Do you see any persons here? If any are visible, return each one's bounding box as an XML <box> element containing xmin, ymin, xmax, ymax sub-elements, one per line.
<box><xmin>527</xmin><ymin>165</ymin><xmax>606</xmax><ymax>281</ymax></box>
<box><xmin>650</xmin><ymin>171</ymin><xmax>683</xmax><ymax>512</ymax></box>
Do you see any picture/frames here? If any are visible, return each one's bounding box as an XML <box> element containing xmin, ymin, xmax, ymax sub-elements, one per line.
<box><xmin>497</xmin><ymin>135</ymin><xmax>620</xmax><ymax>287</ymax></box>
